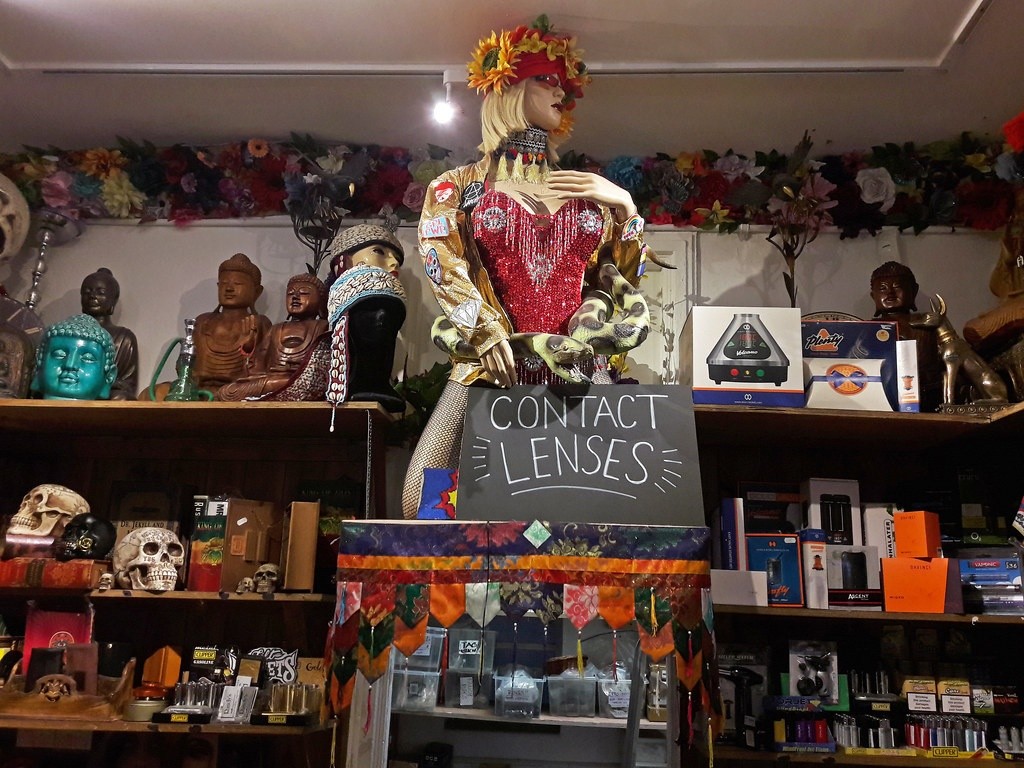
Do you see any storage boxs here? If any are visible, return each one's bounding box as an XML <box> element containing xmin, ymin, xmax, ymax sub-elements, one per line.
<box><xmin>709</xmin><ymin>478</ymin><xmax>964</xmax><ymax>614</ymax></box>
<box><xmin>392</xmin><ymin>628</ymin><xmax>667</xmax><ymax>722</ymax></box>
<box><xmin>881</xmin><ymin>625</ymin><xmax>1021</xmax><ymax>715</ymax></box>
<box><xmin>679</xmin><ymin>306</ymin><xmax>920</xmax><ymax>414</ymax></box>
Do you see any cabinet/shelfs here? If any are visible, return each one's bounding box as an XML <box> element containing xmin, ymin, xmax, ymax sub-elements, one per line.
<box><xmin>0</xmin><ymin>398</ymin><xmax>398</xmax><ymax>768</ymax></box>
<box><xmin>690</xmin><ymin>403</ymin><xmax>1024</xmax><ymax>768</ymax></box>
<box><xmin>318</xmin><ymin>520</ymin><xmax>724</xmax><ymax>768</ymax></box>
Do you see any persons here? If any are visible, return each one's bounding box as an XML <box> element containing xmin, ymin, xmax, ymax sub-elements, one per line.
<box><xmin>870</xmin><ymin>261</ymin><xmax>938</xmax><ymax>413</ymax></box>
<box><xmin>138</xmin><ymin>253</ymin><xmax>327</xmax><ymax>401</ymax></box>
<box><xmin>401</xmin><ymin>13</ymin><xmax>677</xmax><ymax>520</ymax></box>
<box><xmin>329</xmin><ymin>225</ymin><xmax>405</xmax><ymax>286</ymax></box>
<box><xmin>30</xmin><ymin>267</ymin><xmax>138</xmax><ymax>401</ymax></box>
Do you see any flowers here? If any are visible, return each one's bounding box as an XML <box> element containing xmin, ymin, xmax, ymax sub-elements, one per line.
<box><xmin>465</xmin><ymin>13</ymin><xmax>591</xmax><ymax>136</ymax></box>
<box><xmin>0</xmin><ymin>115</ymin><xmax>1024</xmax><ymax>243</ymax></box>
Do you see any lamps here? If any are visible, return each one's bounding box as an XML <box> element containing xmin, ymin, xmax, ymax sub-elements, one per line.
<box><xmin>432</xmin><ymin>70</ymin><xmax>468</xmax><ymax>124</ymax></box>
